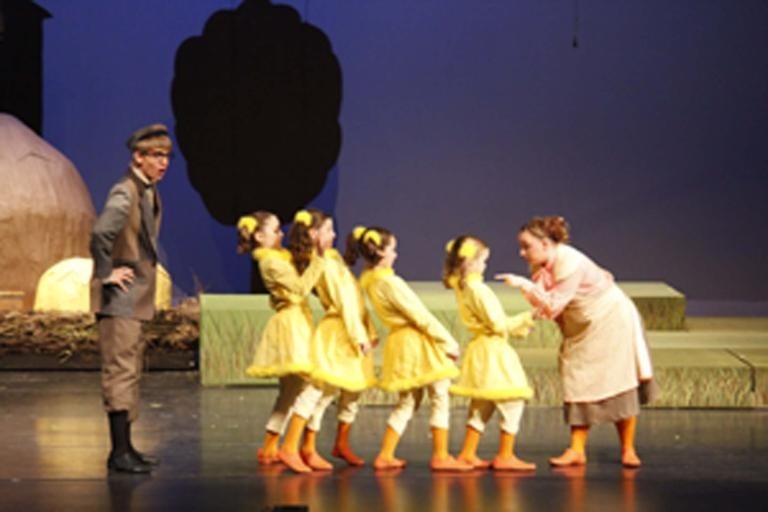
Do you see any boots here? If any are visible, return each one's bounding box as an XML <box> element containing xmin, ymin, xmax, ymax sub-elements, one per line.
<box><xmin>127</xmin><ymin>423</ymin><xmax>158</xmax><ymax>467</ymax></box>
<box><xmin>105</xmin><ymin>410</ymin><xmax>153</xmax><ymax>475</ymax></box>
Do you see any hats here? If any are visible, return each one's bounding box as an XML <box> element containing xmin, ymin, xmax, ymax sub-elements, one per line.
<box><xmin>124</xmin><ymin>124</ymin><xmax>171</xmax><ymax>150</ymax></box>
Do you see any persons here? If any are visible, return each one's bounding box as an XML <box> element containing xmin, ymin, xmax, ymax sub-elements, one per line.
<box><xmin>235</xmin><ymin>211</ymin><xmax>324</xmax><ymax>475</ymax></box>
<box><xmin>288</xmin><ymin>210</ymin><xmax>377</xmax><ymax>470</ymax></box>
<box><xmin>494</xmin><ymin>216</ymin><xmax>654</xmax><ymax>468</ymax></box>
<box><xmin>87</xmin><ymin>122</ymin><xmax>175</xmax><ymax>472</ymax></box>
<box><xmin>345</xmin><ymin>224</ymin><xmax>473</xmax><ymax>474</ymax></box>
<box><xmin>442</xmin><ymin>235</ymin><xmax>541</xmax><ymax>473</ymax></box>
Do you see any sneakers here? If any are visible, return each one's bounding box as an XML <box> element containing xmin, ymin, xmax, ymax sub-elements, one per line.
<box><xmin>620</xmin><ymin>447</ymin><xmax>640</xmax><ymax>466</ymax></box>
<box><xmin>455</xmin><ymin>453</ymin><xmax>493</xmax><ymax>469</ymax></box>
<box><xmin>330</xmin><ymin>445</ymin><xmax>367</xmax><ymax>467</ymax></box>
<box><xmin>371</xmin><ymin>451</ymin><xmax>407</xmax><ymax>469</ymax></box>
<box><xmin>299</xmin><ymin>447</ymin><xmax>333</xmax><ymax>471</ymax></box>
<box><xmin>489</xmin><ymin>456</ymin><xmax>538</xmax><ymax>472</ymax></box>
<box><xmin>276</xmin><ymin>447</ymin><xmax>313</xmax><ymax>473</ymax></box>
<box><xmin>547</xmin><ymin>447</ymin><xmax>588</xmax><ymax>467</ymax></box>
<box><xmin>258</xmin><ymin>449</ymin><xmax>280</xmax><ymax>467</ymax></box>
<box><xmin>430</xmin><ymin>454</ymin><xmax>474</xmax><ymax>474</ymax></box>
